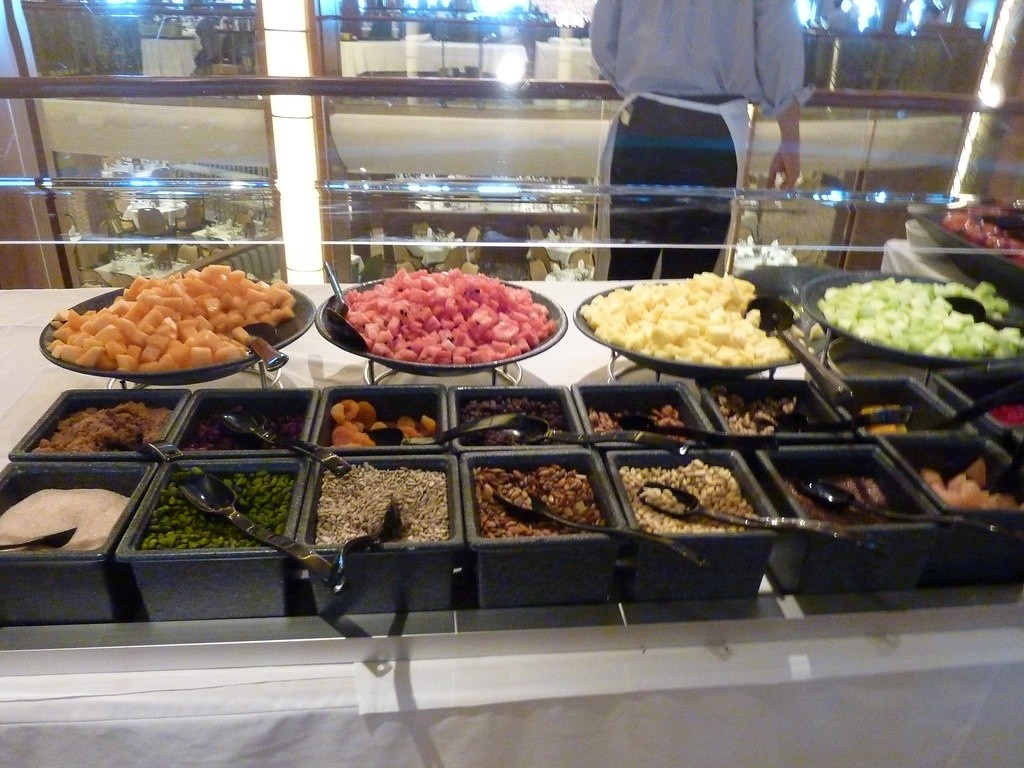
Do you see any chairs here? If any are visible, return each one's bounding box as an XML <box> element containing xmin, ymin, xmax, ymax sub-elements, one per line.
<box><xmin>77</xmin><ymin>212</ymin><xmax>280</xmax><ymax>287</ymax></box>
<box><xmin>389</xmin><ymin>220</ymin><xmax>595</xmax><ymax>281</ymax></box>
<box><xmin>170</xmin><ymin>201</ymin><xmax>204</xmax><ymax>249</ymax></box>
<box><xmin>137</xmin><ymin>208</ymin><xmax>171</xmax><ymax>254</ymax></box>
<box><xmin>105</xmin><ymin>206</ymin><xmax>138</xmax><ymax>252</ymax></box>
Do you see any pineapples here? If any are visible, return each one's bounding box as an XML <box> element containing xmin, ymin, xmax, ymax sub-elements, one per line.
<box><xmin>581</xmin><ymin>271</ymin><xmax>826</xmax><ymax>367</ymax></box>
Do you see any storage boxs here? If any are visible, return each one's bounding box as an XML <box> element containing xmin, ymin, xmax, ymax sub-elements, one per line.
<box><xmin>0</xmin><ymin>459</ymin><xmax>158</xmax><ymax>626</ymax></box>
<box><xmin>570</xmin><ymin>382</ymin><xmax>717</xmax><ymax>459</ymax></box>
<box><xmin>113</xmin><ymin>458</ymin><xmax>311</xmax><ymax>622</ymax></box>
<box><xmin>809</xmin><ymin>368</ymin><xmax>1024</xmax><ymax>588</ymax></box>
<box><xmin>159</xmin><ymin>386</ymin><xmax>321</xmax><ymax>460</ymax></box>
<box><xmin>459</xmin><ymin>448</ymin><xmax>629</xmax><ymax>610</ymax></box>
<box><xmin>697</xmin><ymin>378</ymin><xmax>857</xmax><ymax>450</ymax></box>
<box><xmin>754</xmin><ymin>443</ymin><xmax>944</xmax><ymax>596</ymax></box>
<box><xmin>1</xmin><ymin>389</ymin><xmax>191</xmax><ymax>461</ymax></box>
<box><xmin>604</xmin><ymin>448</ymin><xmax>783</xmax><ymax>604</ymax></box>
<box><xmin>294</xmin><ymin>455</ymin><xmax>467</xmax><ymax>616</ymax></box>
<box><xmin>446</xmin><ymin>386</ymin><xmax>587</xmax><ymax>454</ymax></box>
<box><xmin>309</xmin><ymin>384</ymin><xmax>449</xmax><ymax>456</ymax></box>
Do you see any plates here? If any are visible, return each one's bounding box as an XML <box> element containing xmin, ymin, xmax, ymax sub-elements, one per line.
<box><xmin>910</xmin><ymin>209</ymin><xmax>1024</xmax><ymax>307</ymax></box>
<box><xmin>40</xmin><ymin>280</ymin><xmax>316</xmax><ymax>385</ymax></box>
<box><xmin>573</xmin><ymin>284</ymin><xmax>826</xmax><ymax>378</ymax></box>
<box><xmin>314</xmin><ymin>276</ymin><xmax>567</xmax><ymax>377</ymax></box>
<box><xmin>800</xmin><ymin>271</ymin><xmax>1024</xmax><ymax>366</ymax></box>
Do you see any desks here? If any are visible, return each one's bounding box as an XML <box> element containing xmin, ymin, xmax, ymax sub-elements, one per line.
<box><xmin>140</xmin><ymin>28</ymin><xmax>204</xmax><ymax>77</ymax></box>
<box><xmin>526</xmin><ymin>237</ymin><xmax>590</xmax><ymax>267</ymax></box>
<box><xmin>122</xmin><ymin>199</ymin><xmax>187</xmax><ymax>229</ymax></box>
<box><xmin>402</xmin><ymin>238</ymin><xmax>471</xmax><ymax>272</ymax></box>
<box><xmin>0</xmin><ymin>277</ymin><xmax>1024</xmax><ymax>768</ymax></box>
<box><xmin>533</xmin><ymin>41</ymin><xmax>599</xmax><ymax>80</ymax></box>
<box><xmin>94</xmin><ymin>257</ymin><xmax>187</xmax><ymax>279</ymax></box>
<box><xmin>190</xmin><ymin>224</ymin><xmax>258</xmax><ymax>249</ymax></box>
<box><xmin>732</xmin><ymin>245</ymin><xmax>797</xmax><ymax>277</ymax></box>
<box><xmin>340</xmin><ymin>39</ymin><xmax>443</xmax><ymax>77</ymax></box>
<box><xmin>442</xmin><ymin>41</ymin><xmax>529</xmax><ymax>78</ymax></box>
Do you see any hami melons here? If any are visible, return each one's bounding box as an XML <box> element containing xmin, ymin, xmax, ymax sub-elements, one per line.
<box><xmin>46</xmin><ymin>264</ymin><xmax>297</xmax><ymax>371</ymax></box>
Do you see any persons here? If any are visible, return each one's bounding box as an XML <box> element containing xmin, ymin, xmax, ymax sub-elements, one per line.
<box><xmin>589</xmin><ymin>1</ymin><xmax>817</xmax><ymax>281</ymax></box>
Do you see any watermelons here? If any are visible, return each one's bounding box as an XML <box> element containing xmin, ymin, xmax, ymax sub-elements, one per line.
<box><xmin>343</xmin><ymin>268</ymin><xmax>557</xmax><ymax>365</ymax></box>
